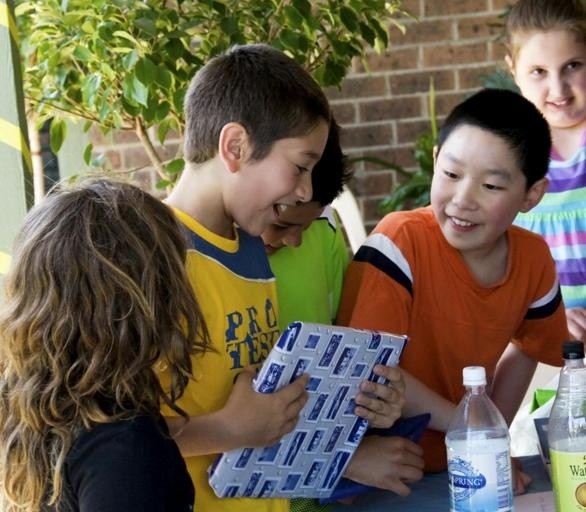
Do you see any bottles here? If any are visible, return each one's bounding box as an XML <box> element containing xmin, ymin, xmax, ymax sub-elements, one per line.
<box><xmin>547</xmin><ymin>341</ymin><xmax>586</xmax><ymax>512</ymax></box>
<box><xmin>445</xmin><ymin>365</ymin><xmax>514</xmax><ymax>512</ymax></box>
